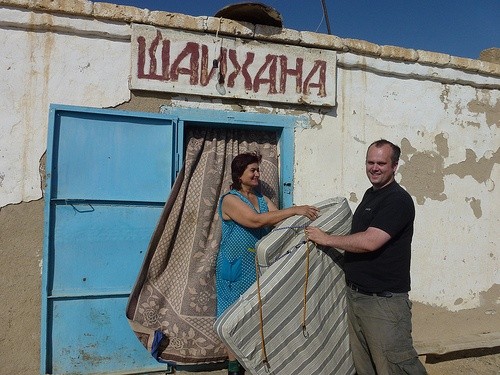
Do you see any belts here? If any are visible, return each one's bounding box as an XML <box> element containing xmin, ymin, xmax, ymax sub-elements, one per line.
<box><xmin>346</xmin><ymin>281</ymin><xmax>407</xmax><ymax>298</ymax></box>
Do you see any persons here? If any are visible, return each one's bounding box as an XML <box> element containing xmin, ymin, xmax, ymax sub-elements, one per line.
<box><xmin>215</xmin><ymin>153</ymin><xmax>320</xmax><ymax>375</ymax></box>
<box><xmin>307</xmin><ymin>140</ymin><xmax>428</xmax><ymax>375</ymax></box>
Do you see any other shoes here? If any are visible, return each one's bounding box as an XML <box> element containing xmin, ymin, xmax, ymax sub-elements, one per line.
<box><xmin>228</xmin><ymin>370</ymin><xmax>244</xmax><ymax>375</ymax></box>
